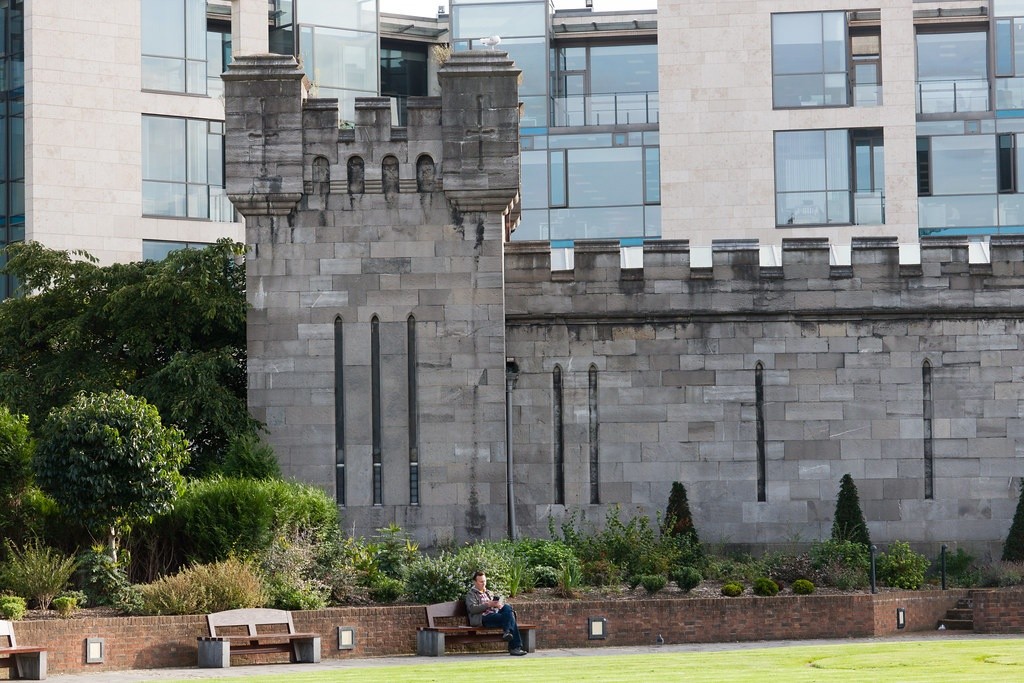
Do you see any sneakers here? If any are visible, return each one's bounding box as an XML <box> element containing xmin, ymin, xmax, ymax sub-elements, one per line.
<box><xmin>509</xmin><ymin>647</ymin><xmax>527</xmax><ymax>655</ymax></box>
<box><xmin>503</xmin><ymin>632</ymin><xmax>512</xmax><ymax>640</ymax></box>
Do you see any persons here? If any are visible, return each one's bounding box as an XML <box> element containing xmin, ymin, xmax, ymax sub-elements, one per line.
<box><xmin>465</xmin><ymin>572</ymin><xmax>528</xmax><ymax>656</ymax></box>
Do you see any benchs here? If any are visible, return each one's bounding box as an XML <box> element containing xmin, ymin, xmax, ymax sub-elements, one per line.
<box><xmin>197</xmin><ymin>608</ymin><xmax>322</xmax><ymax>669</ymax></box>
<box><xmin>0</xmin><ymin>620</ymin><xmax>48</xmax><ymax>680</ymax></box>
<box><xmin>417</xmin><ymin>599</ymin><xmax>537</xmax><ymax>657</ymax></box>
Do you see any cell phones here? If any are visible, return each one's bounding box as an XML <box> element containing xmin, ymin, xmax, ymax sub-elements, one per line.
<box><xmin>492</xmin><ymin>596</ymin><xmax>500</xmax><ymax>602</ymax></box>
